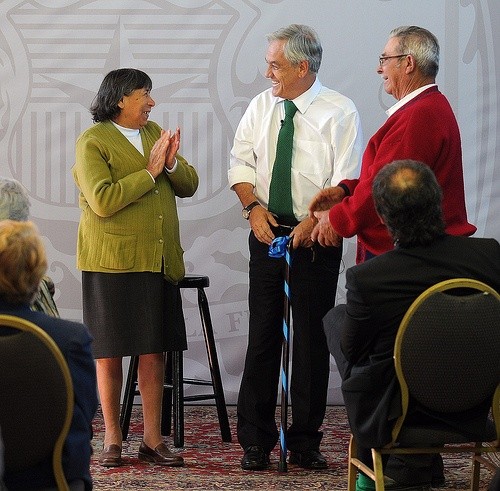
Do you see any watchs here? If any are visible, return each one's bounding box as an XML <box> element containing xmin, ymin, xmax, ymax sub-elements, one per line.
<box><xmin>241</xmin><ymin>200</ymin><xmax>259</xmax><ymax>221</ymax></box>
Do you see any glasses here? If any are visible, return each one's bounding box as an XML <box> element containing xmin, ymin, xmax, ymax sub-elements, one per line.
<box><xmin>379</xmin><ymin>54</ymin><xmax>407</xmax><ymax>65</ymax></box>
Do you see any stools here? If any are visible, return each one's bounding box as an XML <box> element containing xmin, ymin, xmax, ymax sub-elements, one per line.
<box><xmin>120</xmin><ymin>274</ymin><xmax>232</xmax><ymax>449</ymax></box>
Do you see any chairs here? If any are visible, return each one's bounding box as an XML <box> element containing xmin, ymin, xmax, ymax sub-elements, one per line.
<box><xmin>0</xmin><ymin>314</ymin><xmax>75</xmax><ymax>491</ymax></box>
<box><xmin>348</xmin><ymin>279</ymin><xmax>500</xmax><ymax>491</ymax></box>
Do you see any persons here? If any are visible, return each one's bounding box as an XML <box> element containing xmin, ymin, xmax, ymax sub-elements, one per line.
<box><xmin>0</xmin><ymin>177</ymin><xmax>99</xmax><ymax>491</ymax></box>
<box><xmin>308</xmin><ymin>23</ymin><xmax>478</xmax><ymax>261</ymax></box>
<box><xmin>323</xmin><ymin>158</ymin><xmax>499</xmax><ymax>489</ymax></box>
<box><xmin>76</xmin><ymin>67</ymin><xmax>200</xmax><ymax>469</ymax></box>
<box><xmin>226</xmin><ymin>22</ymin><xmax>365</xmax><ymax>473</ymax></box>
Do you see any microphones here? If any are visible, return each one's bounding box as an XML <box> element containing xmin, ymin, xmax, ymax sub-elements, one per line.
<box><xmin>281</xmin><ymin>119</ymin><xmax>284</xmax><ymax>127</ymax></box>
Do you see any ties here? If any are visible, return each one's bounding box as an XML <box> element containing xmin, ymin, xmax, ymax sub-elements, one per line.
<box><xmin>267</xmin><ymin>99</ymin><xmax>297</xmax><ymax>216</ymax></box>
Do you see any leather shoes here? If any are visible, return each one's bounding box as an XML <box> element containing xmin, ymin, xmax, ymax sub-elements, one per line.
<box><xmin>287</xmin><ymin>447</ymin><xmax>328</xmax><ymax>468</ymax></box>
<box><xmin>241</xmin><ymin>444</ymin><xmax>270</xmax><ymax>469</ymax></box>
<box><xmin>138</xmin><ymin>441</ymin><xmax>184</xmax><ymax>466</ymax></box>
<box><xmin>99</xmin><ymin>441</ymin><xmax>122</xmax><ymax>466</ymax></box>
